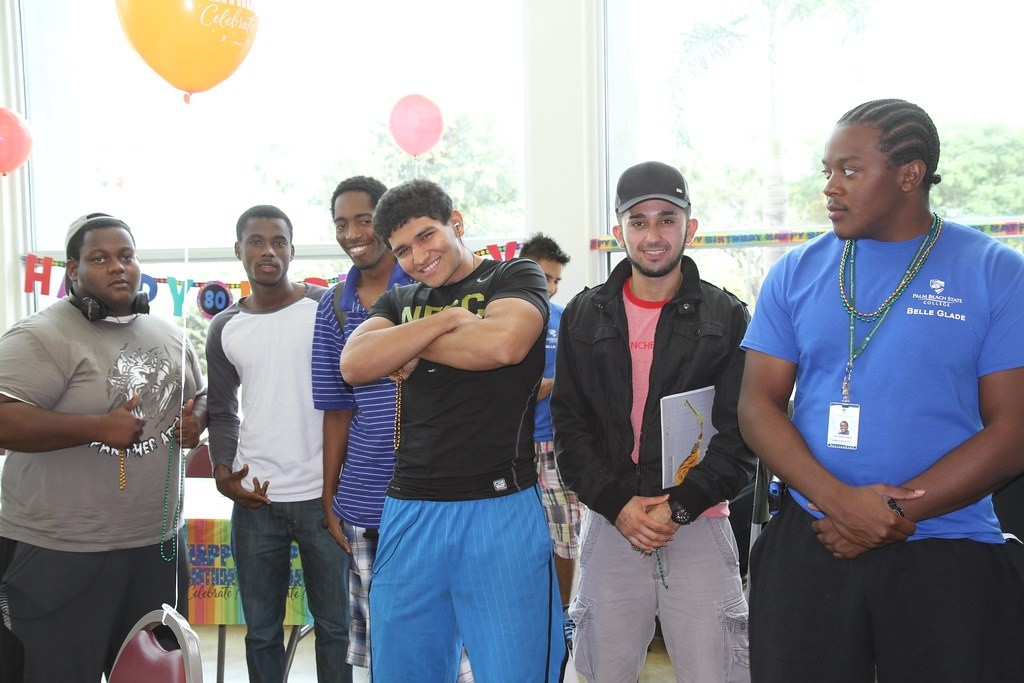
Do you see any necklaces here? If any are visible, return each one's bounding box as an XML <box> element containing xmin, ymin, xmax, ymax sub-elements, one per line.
<box><xmin>391</xmin><ymin>371</ymin><xmax>402</xmax><ymax>448</ymax></box>
<box><xmin>119</xmin><ymin>447</ymin><xmax>126</xmax><ymax>490</ymax></box>
<box><xmin>160</xmin><ymin>427</ymin><xmax>186</xmax><ymax>562</ymax></box>
<box><xmin>839</xmin><ymin>211</ymin><xmax>943</xmax><ymax>323</ymax></box>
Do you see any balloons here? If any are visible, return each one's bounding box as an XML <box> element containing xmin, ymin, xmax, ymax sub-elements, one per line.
<box><xmin>0</xmin><ymin>107</ymin><xmax>33</xmax><ymax>173</ymax></box>
<box><xmin>116</xmin><ymin>0</ymin><xmax>260</xmax><ymax>103</ymax></box>
<box><xmin>390</xmin><ymin>95</ymin><xmax>445</xmax><ymax>157</ymax></box>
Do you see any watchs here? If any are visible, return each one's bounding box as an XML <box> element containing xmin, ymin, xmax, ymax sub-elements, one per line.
<box><xmin>668</xmin><ymin>498</ymin><xmax>691</xmax><ymax>525</ymax></box>
<box><xmin>887</xmin><ymin>498</ymin><xmax>905</xmax><ymax>518</ymax></box>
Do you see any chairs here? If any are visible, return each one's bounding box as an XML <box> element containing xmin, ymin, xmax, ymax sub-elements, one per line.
<box><xmin>108</xmin><ymin>611</ymin><xmax>204</xmax><ymax>683</ymax></box>
<box><xmin>185</xmin><ymin>434</ymin><xmax>214</xmax><ymax>479</ymax></box>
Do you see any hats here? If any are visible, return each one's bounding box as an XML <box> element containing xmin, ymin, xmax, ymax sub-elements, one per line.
<box><xmin>615</xmin><ymin>161</ymin><xmax>691</xmax><ymax>213</ymax></box>
<box><xmin>65</xmin><ymin>213</ymin><xmax>121</xmax><ymax>297</ymax></box>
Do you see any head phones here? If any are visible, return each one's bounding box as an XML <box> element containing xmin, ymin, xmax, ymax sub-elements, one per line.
<box><xmin>69</xmin><ymin>286</ymin><xmax>150</xmax><ymax>322</ymax></box>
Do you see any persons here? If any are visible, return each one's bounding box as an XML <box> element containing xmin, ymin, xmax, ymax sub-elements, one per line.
<box><xmin>311</xmin><ymin>175</ymin><xmax>584</xmax><ymax>683</ymax></box>
<box><xmin>838</xmin><ymin>420</ymin><xmax>849</xmax><ymax>435</ymax></box>
<box><xmin>0</xmin><ymin>212</ymin><xmax>209</xmax><ymax>683</ymax></box>
<box><xmin>550</xmin><ymin>161</ymin><xmax>757</xmax><ymax>683</ymax></box>
<box><xmin>204</xmin><ymin>205</ymin><xmax>354</xmax><ymax>683</ymax></box>
<box><xmin>737</xmin><ymin>99</ymin><xmax>1024</xmax><ymax>683</ymax></box>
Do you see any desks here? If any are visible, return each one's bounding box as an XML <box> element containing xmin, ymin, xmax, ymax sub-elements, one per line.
<box><xmin>184</xmin><ymin>474</ymin><xmax>315</xmax><ymax>682</ymax></box>
